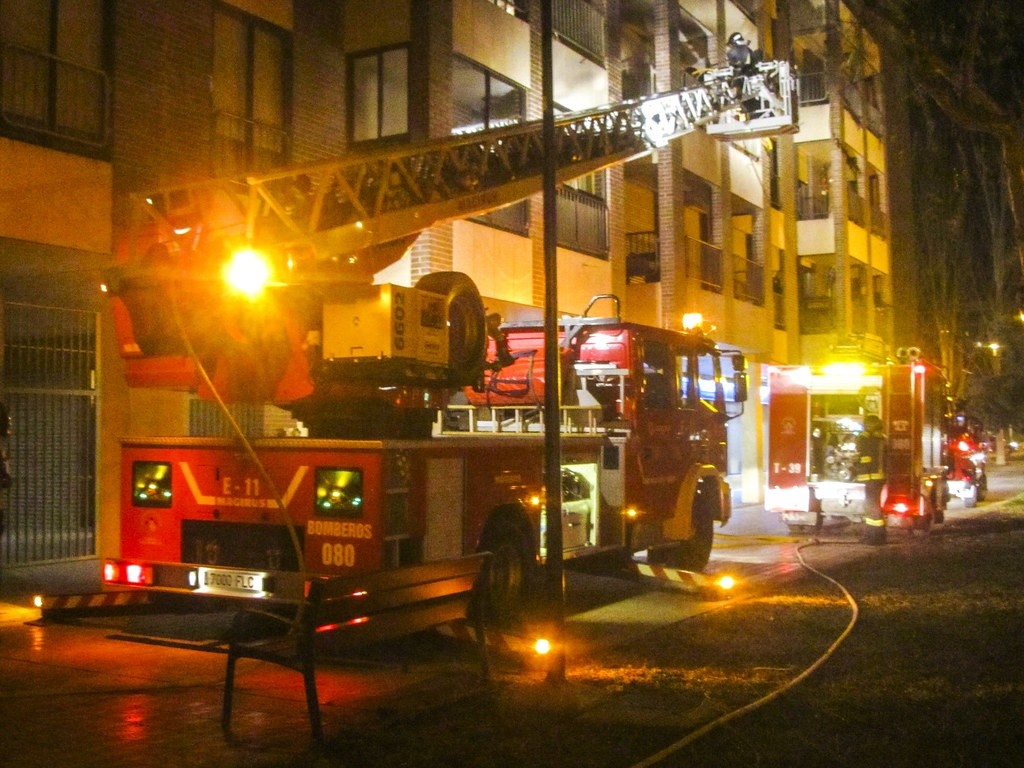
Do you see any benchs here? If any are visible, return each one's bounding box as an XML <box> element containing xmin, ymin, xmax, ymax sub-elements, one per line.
<box><xmin>220</xmin><ymin>551</ymin><xmax>499</xmax><ymax>743</ymax></box>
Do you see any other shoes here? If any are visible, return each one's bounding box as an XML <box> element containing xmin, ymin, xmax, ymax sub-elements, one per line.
<box><xmin>859</xmin><ymin>537</ymin><xmax>887</xmax><ymax>545</ymax></box>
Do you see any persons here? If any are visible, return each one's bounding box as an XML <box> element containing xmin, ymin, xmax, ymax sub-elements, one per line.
<box><xmin>854</xmin><ymin>415</ymin><xmax>890</xmax><ymax>547</ymax></box>
<box><xmin>727</xmin><ymin>32</ymin><xmax>774</xmax><ymax>118</ymax></box>
<box><xmin>1</xmin><ymin>400</ymin><xmax>14</xmax><ymax>533</ymax></box>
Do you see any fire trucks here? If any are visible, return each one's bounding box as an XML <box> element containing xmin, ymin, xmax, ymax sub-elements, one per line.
<box><xmin>762</xmin><ymin>345</ymin><xmax>998</xmax><ymax>541</ymax></box>
<box><xmin>89</xmin><ymin>50</ymin><xmax>802</xmax><ymax>658</ymax></box>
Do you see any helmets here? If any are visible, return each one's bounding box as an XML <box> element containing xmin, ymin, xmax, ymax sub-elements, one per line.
<box><xmin>864</xmin><ymin>415</ymin><xmax>882</xmax><ymax>432</ymax></box>
